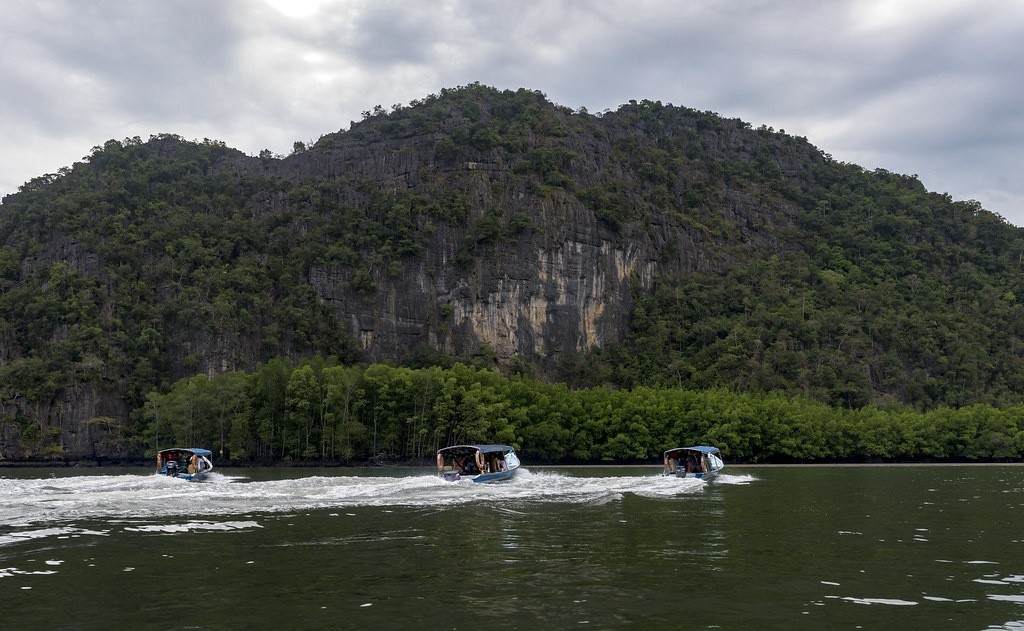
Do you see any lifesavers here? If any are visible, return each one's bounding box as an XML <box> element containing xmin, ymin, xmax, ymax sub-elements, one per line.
<box><xmin>474</xmin><ymin>450</ymin><xmax>485</xmax><ymax>468</ymax></box>
<box><xmin>156</xmin><ymin>454</ymin><xmax>163</xmax><ymax>469</ymax></box>
<box><xmin>700</xmin><ymin>455</ymin><xmax>709</xmax><ymax>472</ymax></box>
<box><xmin>436</xmin><ymin>454</ymin><xmax>446</xmax><ymax>470</ymax></box>
<box><xmin>664</xmin><ymin>456</ymin><xmax>671</xmax><ymax>472</ymax></box>
<box><xmin>191</xmin><ymin>454</ymin><xmax>198</xmax><ymax>468</ymax></box>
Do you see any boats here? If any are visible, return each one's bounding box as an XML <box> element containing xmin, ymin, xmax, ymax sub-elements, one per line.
<box><xmin>156</xmin><ymin>447</ymin><xmax>213</xmax><ymax>483</ymax></box>
<box><xmin>663</xmin><ymin>445</ymin><xmax>725</xmax><ymax>479</ymax></box>
<box><xmin>437</xmin><ymin>445</ymin><xmax>521</xmax><ymax>484</ymax></box>
